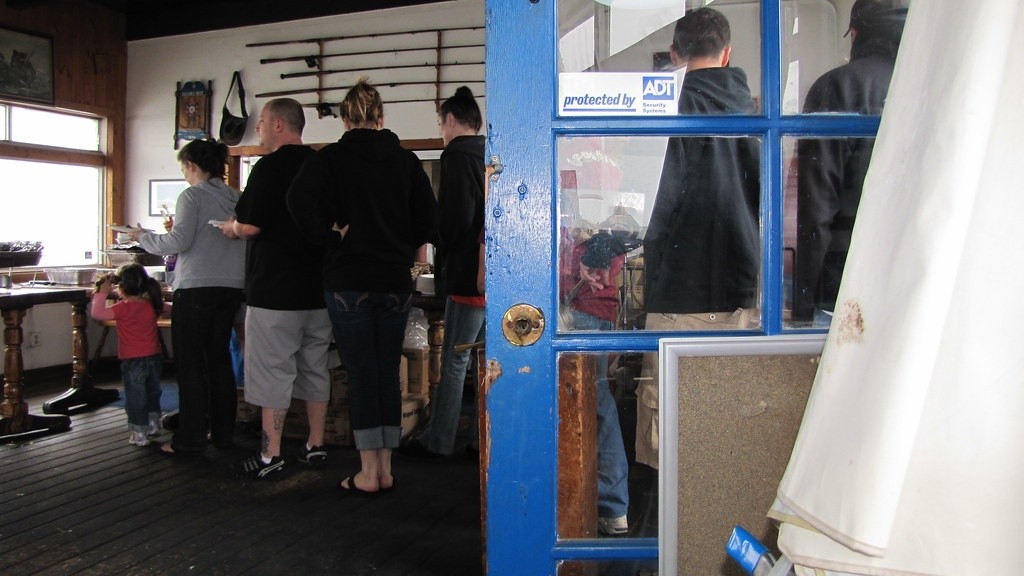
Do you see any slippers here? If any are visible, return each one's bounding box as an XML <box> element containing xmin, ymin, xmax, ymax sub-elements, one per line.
<box><xmin>338</xmin><ymin>472</ymin><xmax>377</xmax><ymax>498</ymax></box>
<box><xmin>380</xmin><ymin>472</ymin><xmax>401</xmax><ymax>495</ymax></box>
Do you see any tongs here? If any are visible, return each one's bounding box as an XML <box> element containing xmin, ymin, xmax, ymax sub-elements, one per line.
<box><xmin>161</xmin><ymin>204</ymin><xmax>174</xmax><ymax>233</ymax></box>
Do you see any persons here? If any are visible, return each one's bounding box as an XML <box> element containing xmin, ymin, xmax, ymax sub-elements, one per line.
<box><xmin>557</xmin><ymin>137</ymin><xmax>629</xmax><ymax>535</ymax></box>
<box><xmin>285</xmin><ymin>77</ymin><xmax>437</xmax><ymax>496</ymax></box>
<box><xmin>790</xmin><ymin>0</ymin><xmax>911</xmax><ymax>329</ymax></box>
<box><xmin>400</xmin><ymin>86</ymin><xmax>484</xmax><ymax>470</ymax></box>
<box><xmin>90</xmin><ymin>261</ymin><xmax>165</xmax><ymax>447</ymax></box>
<box><xmin>579</xmin><ymin>7</ymin><xmax>764</xmax><ymax>467</ymax></box>
<box><xmin>217</xmin><ymin>97</ymin><xmax>334</xmax><ymax>484</ymax></box>
<box><xmin>127</xmin><ymin>138</ymin><xmax>248</xmax><ymax>457</ymax></box>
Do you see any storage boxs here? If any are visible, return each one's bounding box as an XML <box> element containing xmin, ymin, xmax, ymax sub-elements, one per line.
<box><xmin>235</xmin><ymin>345</ymin><xmax>442</xmax><ymax>444</ymax></box>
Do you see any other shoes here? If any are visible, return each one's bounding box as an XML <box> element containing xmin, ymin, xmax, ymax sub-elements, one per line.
<box><xmin>206</xmin><ymin>432</ymin><xmax>229</xmax><ymax>449</ymax></box>
<box><xmin>398</xmin><ymin>439</ymin><xmax>446</xmax><ymax>465</ymax></box>
<box><xmin>598</xmin><ymin>515</ymin><xmax>630</xmax><ymax>536</ymax></box>
<box><xmin>128</xmin><ymin>431</ymin><xmax>150</xmax><ymax>447</ymax></box>
<box><xmin>159</xmin><ymin>441</ymin><xmax>206</xmax><ymax>461</ymax></box>
<box><xmin>467</xmin><ymin>444</ymin><xmax>479</xmax><ymax>459</ymax></box>
<box><xmin>147</xmin><ymin>422</ymin><xmax>162</xmax><ymax>435</ymax></box>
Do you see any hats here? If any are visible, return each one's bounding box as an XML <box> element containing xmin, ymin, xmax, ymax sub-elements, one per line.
<box><xmin>843</xmin><ymin>1</ymin><xmax>912</xmax><ymax>36</ymax></box>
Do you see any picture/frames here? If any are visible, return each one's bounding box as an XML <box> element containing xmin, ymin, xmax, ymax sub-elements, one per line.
<box><xmin>148</xmin><ymin>179</ymin><xmax>190</xmax><ymax>216</ymax></box>
<box><xmin>0</xmin><ymin>23</ymin><xmax>55</xmax><ymax>105</ymax></box>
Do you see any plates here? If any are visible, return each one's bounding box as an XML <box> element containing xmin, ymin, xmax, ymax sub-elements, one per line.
<box><xmin>415</xmin><ymin>274</ymin><xmax>435</xmax><ymax>294</ymax></box>
<box><xmin>208</xmin><ymin>220</ymin><xmax>227</xmax><ymax>227</ymax></box>
<box><xmin>106</xmin><ymin>224</ymin><xmax>155</xmax><ymax>233</ymax></box>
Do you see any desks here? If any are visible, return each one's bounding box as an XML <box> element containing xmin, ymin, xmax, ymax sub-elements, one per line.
<box><xmin>0</xmin><ymin>281</ymin><xmax>171</xmax><ymax>437</ymax></box>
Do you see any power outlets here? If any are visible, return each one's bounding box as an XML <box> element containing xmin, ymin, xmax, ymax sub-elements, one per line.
<box><xmin>30</xmin><ymin>332</ymin><xmax>41</xmax><ymax>347</ymax></box>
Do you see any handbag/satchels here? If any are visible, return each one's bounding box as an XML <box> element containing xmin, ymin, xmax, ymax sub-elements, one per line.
<box><xmin>219</xmin><ymin>71</ymin><xmax>249</xmax><ymax>147</ymax></box>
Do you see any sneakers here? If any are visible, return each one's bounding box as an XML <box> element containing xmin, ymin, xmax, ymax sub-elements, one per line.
<box><xmin>291</xmin><ymin>441</ymin><xmax>329</xmax><ymax>471</ymax></box>
<box><xmin>234</xmin><ymin>451</ymin><xmax>285</xmax><ymax>481</ymax></box>
